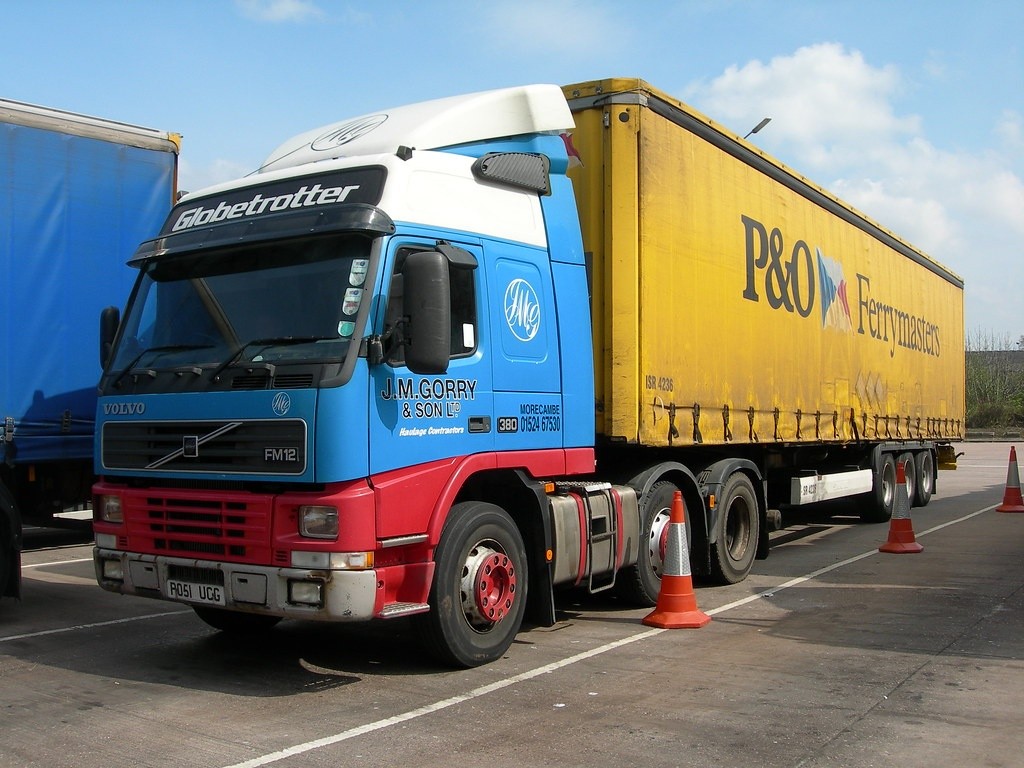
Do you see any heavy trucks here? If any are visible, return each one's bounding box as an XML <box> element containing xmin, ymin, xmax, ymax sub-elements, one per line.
<box><xmin>0</xmin><ymin>96</ymin><xmax>182</xmax><ymax>599</ymax></box>
<box><xmin>86</xmin><ymin>78</ymin><xmax>967</xmax><ymax>666</ymax></box>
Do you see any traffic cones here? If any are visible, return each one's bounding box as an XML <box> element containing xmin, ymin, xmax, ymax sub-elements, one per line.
<box><xmin>879</xmin><ymin>462</ymin><xmax>925</xmax><ymax>555</ymax></box>
<box><xmin>995</xmin><ymin>446</ymin><xmax>1024</xmax><ymax>512</ymax></box>
<box><xmin>642</xmin><ymin>491</ymin><xmax>712</xmax><ymax>629</ymax></box>
